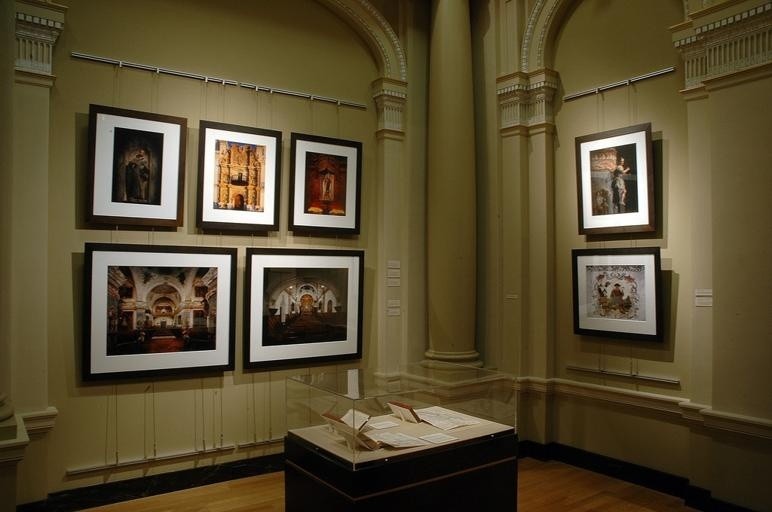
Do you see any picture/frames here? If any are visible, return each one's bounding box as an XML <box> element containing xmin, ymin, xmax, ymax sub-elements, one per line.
<box><xmin>242</xmin><ymin>247</ymin><xmax>365</xmax><ymax>370</ymax></box>
<box><xmin>288</xmin><ymin>132</ymin><xmax>363</xmax><ymax>235</ymax></box>
<box><xmin>196</xmin><ymin>120</ymin><xmax>282</xmax><ymax>232</ymax></box>
<box><xmin>575</xmin><ymin>122</ymin><xmax>657</xmax><ymax>236</ymax></box>
<box><xmin>83</xmin><ymin>101</ymin><xmax>187</xmax><ymax>227</ymax></box>
<box><xmin>570</xmin><ymin>246</ymin><xmax>664</xmax><ymax>343</ymax></box>
<box><xmin>83</xmin><ymin>241</ymin><xmax>237</xmax><ymax>384</ymax></box>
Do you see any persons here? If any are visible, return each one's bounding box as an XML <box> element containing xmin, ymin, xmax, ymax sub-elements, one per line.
<box><xmin>612</xmin><ymin>155</ymin><xmax>630</xmax><ymax>205</ymax></box>
<box><xmin>133</xmin><ymin>149</ymin><xmax>147</xmax><ymax>169</ymax></box>
<box><xmin>594</xmin><ymin>191</ymin><xmax>610</xmax><ymax>214</ymax></box>
